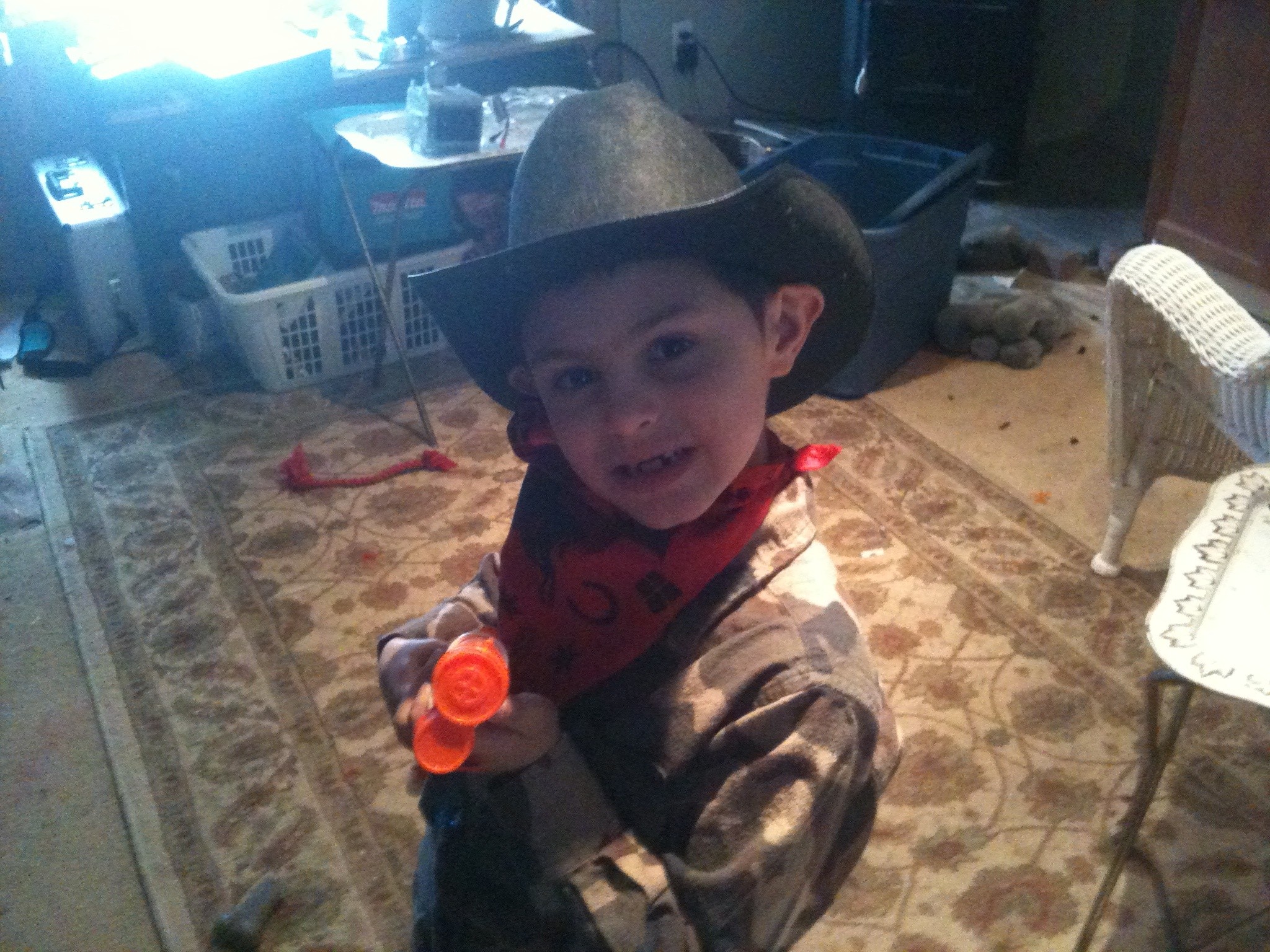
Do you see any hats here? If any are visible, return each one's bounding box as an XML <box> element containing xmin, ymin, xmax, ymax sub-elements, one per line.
<box><xmin>410</xmin><ymin>80</ymin><xmax>880</xmax><ymax>424</ymax></box>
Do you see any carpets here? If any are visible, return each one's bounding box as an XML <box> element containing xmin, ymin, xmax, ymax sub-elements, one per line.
<box><xmin>2</xmin><ymin>277</ymin><xmax>1268</xmax><ymax>952</ymax></box>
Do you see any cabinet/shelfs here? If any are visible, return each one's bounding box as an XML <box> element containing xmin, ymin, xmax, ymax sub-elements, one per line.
<box><xmin>1136</xmin><ymin>3</ymin><xmax>1270</xmax><ymax>292</ymax></box>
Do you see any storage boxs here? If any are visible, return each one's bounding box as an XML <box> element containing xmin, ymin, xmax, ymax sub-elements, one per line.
<box><xmin>726</xmin><ymin>129</ymin><xmax>972</xmax><ymax>404</ymax></box>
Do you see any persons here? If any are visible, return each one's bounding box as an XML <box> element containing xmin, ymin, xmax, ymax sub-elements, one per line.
<box><xmin>377</xmin><ymin>81</ymin><xmax>899</xmax><ymax>951</ymax></box>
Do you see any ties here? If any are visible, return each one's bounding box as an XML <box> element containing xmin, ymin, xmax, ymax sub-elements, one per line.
<box><xmin>465</xmin><ymin>406</ymin><xmax>804</xmax><ymax>771</ymax></box>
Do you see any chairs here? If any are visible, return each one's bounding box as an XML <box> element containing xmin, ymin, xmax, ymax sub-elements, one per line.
<box><xmin>1063</xmin><ymin>465</ymin><xmax>1269</xmax><ymax>952</ymax></box>
<box><xmin>1089</xmin><ymin>245</ymin><xmax>1270</xmax><ymax>589</ymax></box>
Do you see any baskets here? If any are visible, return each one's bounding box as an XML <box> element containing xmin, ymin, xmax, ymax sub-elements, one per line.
<box><xmin>180</xmin><ymin>215</ymin><xmax>478</xmax><ymax>392</ymax></box>
